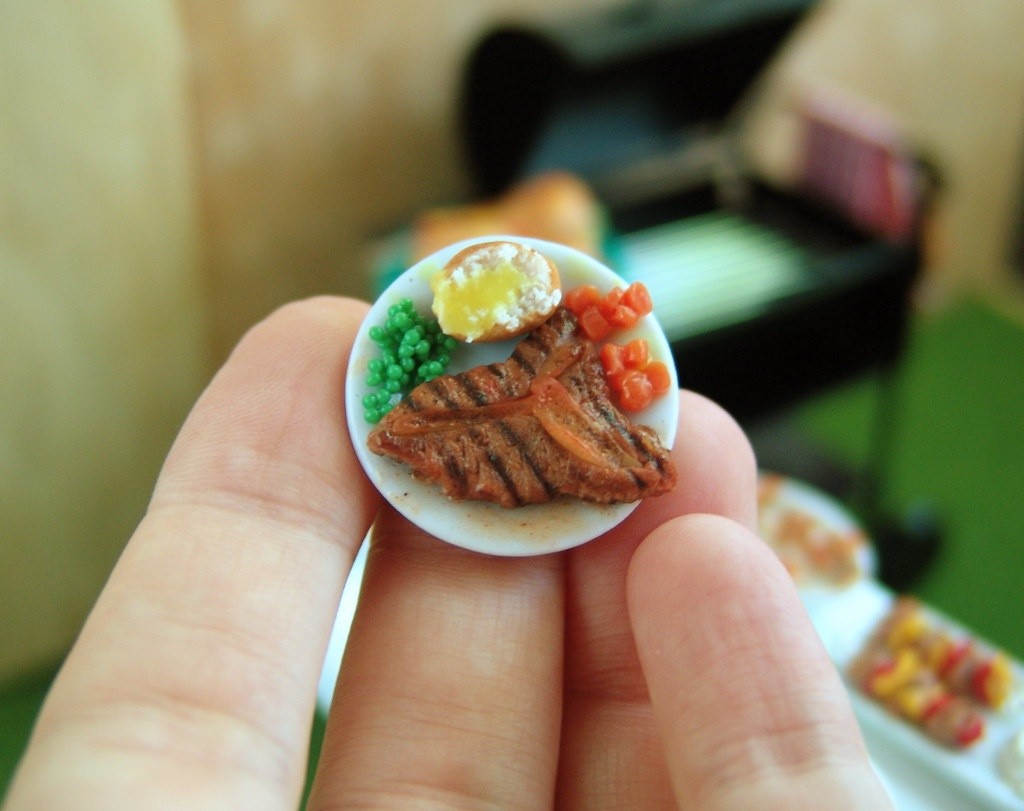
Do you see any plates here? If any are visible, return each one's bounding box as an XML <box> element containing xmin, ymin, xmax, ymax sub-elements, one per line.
<box><xmin>346</xmin><ymin>236</ymin><xmax>680</xmax><ymax>558</ymax></box>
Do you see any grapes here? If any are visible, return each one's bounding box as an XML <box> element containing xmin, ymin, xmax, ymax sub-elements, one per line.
<box><xmin>363</xmin><ymin>301</ymin><xmax>456</xmax><ymax>422</ymax></box>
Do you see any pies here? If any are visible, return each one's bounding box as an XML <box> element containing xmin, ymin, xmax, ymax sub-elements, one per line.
<box><xmin>368</xmin><ymin>306</ymin><xmax>677</xmax><ymax>506</ymax></box>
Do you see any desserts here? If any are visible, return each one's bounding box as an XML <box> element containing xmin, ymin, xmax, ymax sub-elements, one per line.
<box><xmin>434</xmin><ymin>242</ymin><xmax>563</xmax><ymax>340</ymax></box>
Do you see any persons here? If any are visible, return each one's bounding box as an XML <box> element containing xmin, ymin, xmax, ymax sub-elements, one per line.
<box><xmin>0</xmin><ymin>292</ymin><xmax>901</xmax><ymax>811</ymax></box>
